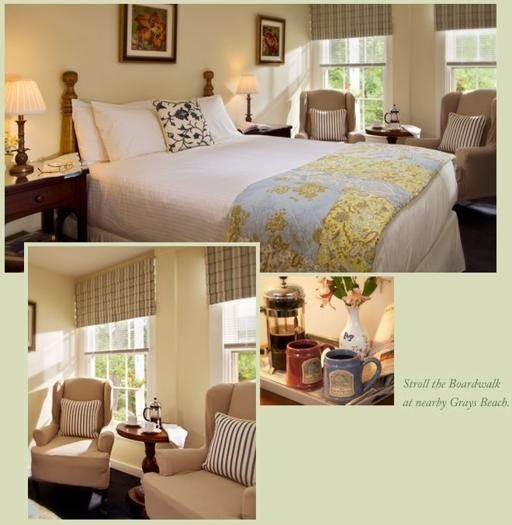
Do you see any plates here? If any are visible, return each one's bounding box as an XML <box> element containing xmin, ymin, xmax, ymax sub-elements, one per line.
<box><xmin>125</xmin><ymin>421</ymin><xmax>142</xmax><ymax>426</ymax></box>
<box><xmin>142</xmin><ymin>429</ymin><xmax>161</xmax><ymax>434</ymax></box>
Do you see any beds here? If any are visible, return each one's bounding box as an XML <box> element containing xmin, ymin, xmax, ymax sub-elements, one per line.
<box><xmin>62</xmin><ymin>71</ymin><xmax>466</xmax><ymax>272</ymax></box>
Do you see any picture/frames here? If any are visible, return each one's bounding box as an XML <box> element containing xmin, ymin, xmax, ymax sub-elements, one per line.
<box><xmin>28</xmin><ymin>301</ymin><xmax>36</xmax><ymax>352</ymax></box>
<box><xmin>258</xmin><ymin>15</ymin><xmax>285</xmax><ymax>63</ymax></box>
<box><xmin>124</xmin><ymin>4</ymin><xmax>178</xmax><ymax>63</ymax></box>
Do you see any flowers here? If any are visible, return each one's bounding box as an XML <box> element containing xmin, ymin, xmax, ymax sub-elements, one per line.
<box><xmin>314</xmin><ymin>276</ymin><xmax>391</xmax><ymax>309</ymax></box>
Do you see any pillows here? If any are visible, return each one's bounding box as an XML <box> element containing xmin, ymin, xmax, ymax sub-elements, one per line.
<box><xmin>201</xmin><ymin>412</ymin><xmax>256</xmax><ymax>486</ymax></box>
<box><xmin>60</xmin><ymin>398</ymin><xmax>102</xmax><ymax>438</ymax></box>
<box><xmin>437</xmin><ymin>111</ymin><xmax>487</xmax><ymax>151</ymax></box>
<box><xmin>309</xmin><ymin>107</ymin><xmax>347</xmax><ymax>140</ymax></box>
<box><xmin>71</xmin><ymin>93</ymin><xmax>241</xmax><ymax>163</ymax></box>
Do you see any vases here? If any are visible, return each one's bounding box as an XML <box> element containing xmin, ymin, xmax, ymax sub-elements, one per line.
<box><xmin>340</xmin><ymin>307</ymin><xmax>369</xmax><ymax>357</ymax></box>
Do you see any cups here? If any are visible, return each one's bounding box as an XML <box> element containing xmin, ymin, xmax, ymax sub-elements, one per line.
<box><xmin>127</xmin><ymin>416</ymin><xmax>137</xmax><ymax>424</ymax></box>
<box><xmin>285</xmin><ymin>341</ymin><xmax>336</xmax><ymax>393</ymax></box>
<box><xmin>144</xmin><ymin>422</ymin><xmax>160</xmax><ymax>432</ymax></box>
<box><xmin>320</xmin><ymin>349</ymin><xmax>383</xmax><ymax>403</ymax></box>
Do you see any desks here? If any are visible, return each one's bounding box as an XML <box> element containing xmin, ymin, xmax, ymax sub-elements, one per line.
<box><xmin>260</xmin><ymin>334</ymin><xmax>379</xmax><ymax>405</ymax></box>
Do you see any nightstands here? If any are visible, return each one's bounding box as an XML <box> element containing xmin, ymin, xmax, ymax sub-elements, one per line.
<box><xmin>238</xmin><ymin>124</ymin><xmax>293</xmax><ymax>138</ymax></box>
<box><xmin>5</xmin><ymin>161</ymin><xmax>90</xmax><ymax>242</ymax></box>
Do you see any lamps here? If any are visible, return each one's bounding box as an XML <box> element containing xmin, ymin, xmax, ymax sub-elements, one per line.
<box><xmin>235</xmin><ymin>75</ymin><xmax>258</xmax><ymax>122</ymax></box>
<box><xmin>5</xmin><ymin>81</ymin><xmax>46</xmax><ymax>174</ymax></box>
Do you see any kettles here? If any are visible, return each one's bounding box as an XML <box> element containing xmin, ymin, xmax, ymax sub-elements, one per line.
<box><xmin>260</xmin><ymin>275</ymin><xmax>306</xmax><ymax>373</ymax></box>
<box><xmin>144</xmin><ymin>397</ymin><xmax>162</xmax><ymax>428</ymax></box>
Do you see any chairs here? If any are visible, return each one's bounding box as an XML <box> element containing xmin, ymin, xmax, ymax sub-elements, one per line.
<box><xmin>30</xmin><ymin>378</ymin><xmax>114</xmax><ymax>489</ymax></box>
<box><xmin>294</xmin><ymin>89</ymin><xmax>365</xmax><ymax>143</ymax></box>
<box><xmin>142</xmin><ymin>383</ymin><xmax>256</xmax><ymax>519</ymax></box>
<box><xmin>405</xmin><ymin>89</ymin><xmax>496</xmax><ymax>203</ymax></box>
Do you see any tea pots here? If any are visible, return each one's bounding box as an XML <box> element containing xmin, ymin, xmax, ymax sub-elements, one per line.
<box><xmin>384</xmin><ymin>104</ymin><xmax>400</xmax><ymax>128</ymax></box>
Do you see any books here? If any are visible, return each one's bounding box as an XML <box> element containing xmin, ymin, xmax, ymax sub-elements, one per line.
<box><xmin>238</xmin><ymin>122</ymin><xmax>275</xmax><ymax>133</ymax></box>
